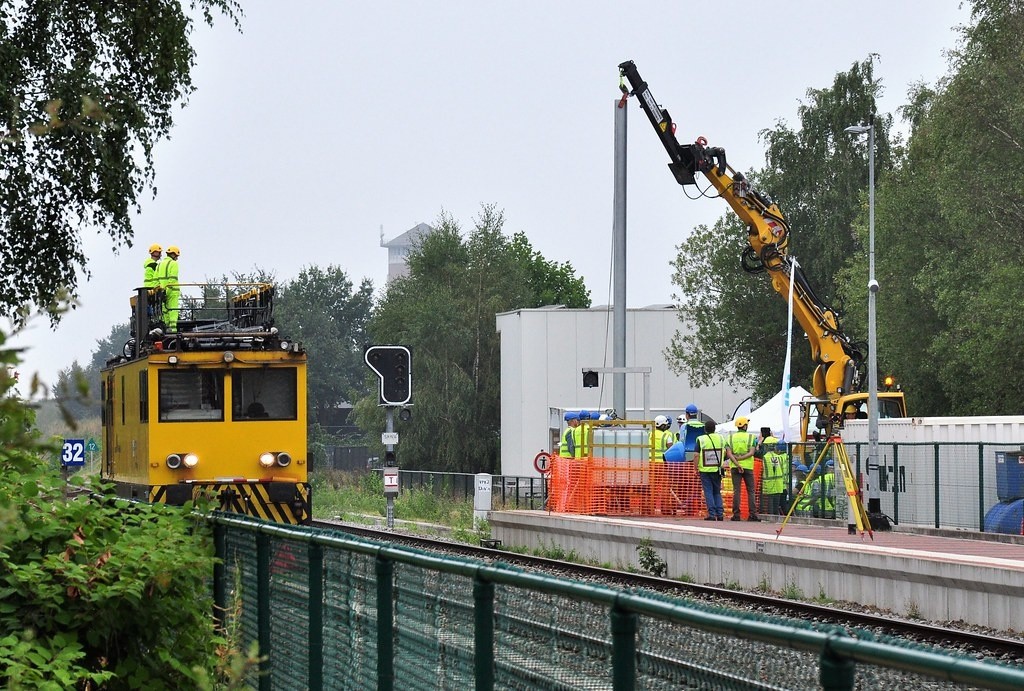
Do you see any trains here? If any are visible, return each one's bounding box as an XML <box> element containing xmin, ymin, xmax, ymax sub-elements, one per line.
<box><xmin>100</xmin><ymin>283</ymin><xmax>315</xmax><ymax>527</ymax></box>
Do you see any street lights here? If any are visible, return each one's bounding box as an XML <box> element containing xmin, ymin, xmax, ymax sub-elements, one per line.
<box><xmin>841</xmin><ymin>122</ymin><xmax>891</xmax><ymax>531</ymax></box>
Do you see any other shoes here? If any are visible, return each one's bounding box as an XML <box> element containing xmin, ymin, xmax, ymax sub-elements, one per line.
<box><xmin>717</xmin><ymin>517</ymin><xmax>723</xmax><ymax>521</ymax></box>
<box><xmin>748</xmin><ymin>516</ymin><xmax>761</xmax><ymax>522</ymax></box>
<box><xmin>731</xmin><ymin>516</ymin><xmax>740</xmax><ymax>521</ymax></box>
<box><xmin>704</xmin><ymin>516</ymin><xmax>716</xmax><ymax>521</ymax></box>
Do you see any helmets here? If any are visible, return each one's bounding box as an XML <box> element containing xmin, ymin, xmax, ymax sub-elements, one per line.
<box><xmin>845</xmin><ymin>405</ymin><xmax>858</xmax><ymax>413</ymax></box>
<box><xmin>735</xmin><ymin>417</ymin><xmax>749</xmax><ymax>427</ymax></box>
<box><xmin>777</xmin><ymin>440</ymin><xmax>787</xmax><ymax>450</ymax></box>
<box><xmin>149</xmin><ymin>244</ymin><xmax>162</xmax><ymax>254</ymax></box>
<box><xmin>655</xmin><ymin>415</ymin><xmax>668</xmax><ymax>427</ymax></box>
<box><xmin>685</xmin><ymin>404</ymin><xmax>698</xmax><ymax>415</ymax></box>
<box><xmin>792</xmin><ymin>459</ymin><xmax>833</xmax><ymax>472</ymax></box>
<box><xmin>565</xmin><ymin>410</ymin><xmax>613</xmax><ymax>426</ymax></box>
<box><xmin>666</xmin><ymin>415</ymin><xmax>672</xmax><ymax>424</ymax></box>
<box><xmin>763</xmin><ymin>437</ymin><xmax>776</xmax><ymax>444</ymax></box>
<box><xmin>166</xmin><ymin>246</ymin><xmax>179</xmax><ymax>256</ymax></box>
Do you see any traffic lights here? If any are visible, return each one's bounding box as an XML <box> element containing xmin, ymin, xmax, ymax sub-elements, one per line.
<box><xmin>363</xmin><ymin>343</ymin><xmax>415</xmax><ymax>407</ymax></box>
<box><xmin>398</xmin><ymin>408</ymin><xmax>411</xmax><ymax>421</ymax></box>
<box><xmin>386</xmin><ymin>450</ymin><xmax>396</xmax><ymax>462</ymax></box>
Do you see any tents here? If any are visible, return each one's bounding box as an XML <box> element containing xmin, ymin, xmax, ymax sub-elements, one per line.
<box><xmin>714</xmin><ymin>386</ymin><xmax>826</xmax><ymax>442</ymax></box>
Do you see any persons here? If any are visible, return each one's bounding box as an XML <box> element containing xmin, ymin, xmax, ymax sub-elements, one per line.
<box><xmin>754</xmin><ymin>430</ymin><xmax>789</xmax><ymax>515</ymax></box>
<box><xmin>144</xmin><ymin>244</ymin><xmax>163</xmax><ymax>287</ymax></box>
<box><xmin>725</xmin><ymin>417</ymin><xmax>761</xmax><ymax>522</ymax></box>
<box><xmin>845</xmin><ymin>405</ymin><xmax>857</xmax><ymax>419</ymax></box>
<box><xmin>694</xmin><ymin>422</ymin><xmax>725</xmax><ymax>521</ymax></box>
<box><xmin>561</xmin><ymin>410</ymin><xmax>600</xmax><ymax>457</ymax></box>
<box><xmin>792</xmin><ymin>459</ymin><xmax>834</xmax><ymax>517</ymax></box>
<box><xmin>153</xmin><ymin>246</ymin><xmax>180</xmax><ymax>333</ymax></box>
<box><xmin>650</xmin><ymin>405</ymin><xmax>705</xmax><ymax>515</ymax></box>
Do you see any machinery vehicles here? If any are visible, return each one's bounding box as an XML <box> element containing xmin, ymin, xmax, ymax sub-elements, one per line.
<box><xmin>614</xmin><ymin>59</ymin><xmax>907</xmax><ymax>481</ymax></box>
